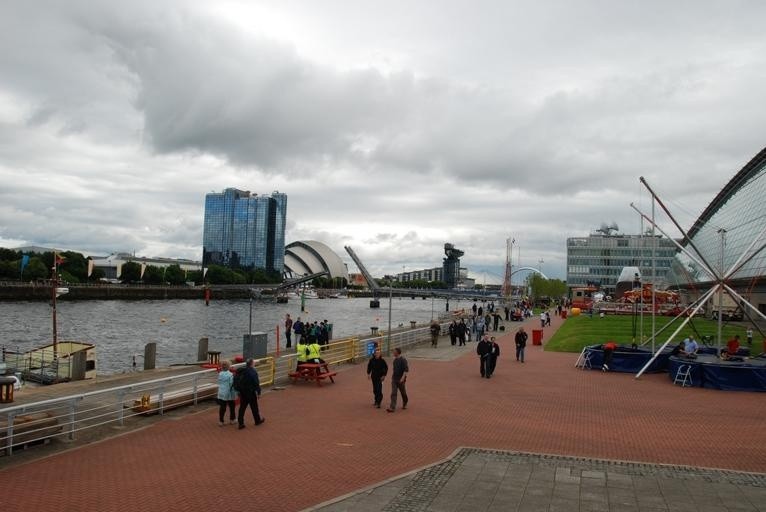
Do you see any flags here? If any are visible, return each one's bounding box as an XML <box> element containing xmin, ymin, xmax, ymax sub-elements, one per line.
<box><xmin>51</xmin><ymin>253</ymin><xmax>65</xmax><ymax>268</ymax></box>
<box><xmin>19</xmin><ymin>254</ymin><xmax>30</xmax><ymax>273</ymax></box>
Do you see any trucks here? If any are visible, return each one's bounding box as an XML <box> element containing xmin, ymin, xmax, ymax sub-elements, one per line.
<box><xmin>709</xmin><ymin>289</ymin><xmax>746</xmax><ymax>322</ymax></box>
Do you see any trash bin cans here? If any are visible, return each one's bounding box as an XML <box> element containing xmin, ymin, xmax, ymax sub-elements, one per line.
<box><xmin>532</xmin><ymin>328</ymin><xmax>543</xmax><ymax>346</ymax></box>
<box><xmin>371</xmin><ymin>326</ymin><xmax>379</xmax><ymax>336</ymax></box>
<box><xmin>0</xmin><ymin>378</ymin><xmax>16</xmax><ymax>402</ymax></box>
<box><xmin>411</xmin><ymin>321</ymin><xmax>417</xmax><ymax>328</ymax></box>
<box><xmin>209</xmin><ymin>352</ymin><xmax>221</xmax><ymax>365</ymax></box>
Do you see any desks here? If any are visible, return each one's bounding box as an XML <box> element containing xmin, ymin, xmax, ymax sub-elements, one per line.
<box><xmin>298</xmin><ymin>360</ymin><xmax>329</xmax><ymax>385</ymax></box>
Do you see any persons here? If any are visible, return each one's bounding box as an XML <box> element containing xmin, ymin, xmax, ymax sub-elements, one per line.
<box><xmin>294</xmin><ymin>336</ymin><xmax>308</xmax><ymax>371</ymax></box>
<box><xmin>233</xmin><ymin>356</ymin><xmax>266</xmax><ymax>430</ymax></box>
<box><xmin>306</xmin><ymin>335</ymin><xmax>325</xmax><ymax>364</ymax></box>
<box><xmin>283</xmin><ymin>313</ymin><xmax>332</xmax><ymax>353</ymax></box>
<box><xmin>366</xmin><ymin>345</ymin><xmax>389</xmax><ymax>409</ymax></box>
<box><xmin>428</xmin><ymin>290</ymin><xmax>578</xmax><ymax>381</ymax></box>
<box><xmin>215</xmin><ymin>358</ymin><xmax>238</xmax><ymax>427</ymax></box>
<box><xmin>670</xmin><ymin>341</ymin><xmax>693</xmax><ymax>358</ymax></box>
<box><xmin>726</xmin><ymin>334</ymin><xmax>741</xmax><ymax>355</ymax></box>
<box><xmin>383</xmin><ymin>347</ymin><xmax>410</xmax><ymax>413</ymax></box>
<box><xmin>682</xmin><ymin>335</ymin><xmax>699</xmax><ymax>355</ymax></box>
<box><xmin>746</xmin><ymin>324</ymin><xmax>753</xmax><ymax>345</ymax></box>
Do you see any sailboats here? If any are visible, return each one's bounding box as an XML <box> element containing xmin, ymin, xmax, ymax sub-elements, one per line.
<box><xmin>0</xmin><ymin>249</ymin><xmax>96</xmax><ymax>389</ymax></box>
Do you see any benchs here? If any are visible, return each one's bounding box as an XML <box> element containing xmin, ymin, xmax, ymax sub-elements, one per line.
<box><xmin>287</xmin><ymin>370</ymin><xmax>301</xmax><ymax>383</ymax></box>
<box><xmin>318</xmin><ymin>371</ymin><xmax>337</xmax><ymax>386</ymax></box>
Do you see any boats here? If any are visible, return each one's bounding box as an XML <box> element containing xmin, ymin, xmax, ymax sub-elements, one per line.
<box><xmin>330</xmin><ymin>293</ymin><xmax>348</xmax><ymax>299</ymax></box>
<box><xmin>296</xmin><ymin>285</ymin><xmax>319</xmax><ymax>299</ymax></box>
<box><xmin>251</xmin><ymin>286</ymin><xmax>289</xmax><ymax>304</ymax></box>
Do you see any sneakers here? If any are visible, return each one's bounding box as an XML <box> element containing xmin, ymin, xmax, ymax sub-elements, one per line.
<box><xmin>218</xmin><ymin>417</ymin><xmax>265</xmax><ymax>430</ymax></box>
<box><xmin>371</xmin><ymin>403</ymin><xmax>408</xmax><ymax>413</ymax></box>
